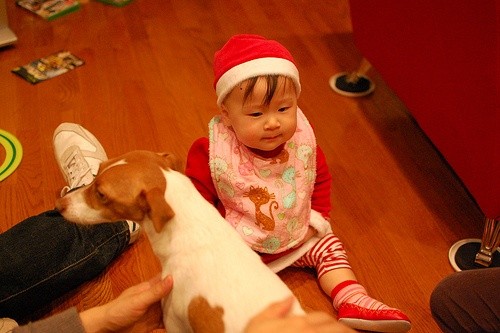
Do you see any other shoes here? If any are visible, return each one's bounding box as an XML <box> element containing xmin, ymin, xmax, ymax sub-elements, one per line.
<box><xmin>337</xmin><ymin>302</ymin><xmax>412</xmax><ymax>333</ymax></box>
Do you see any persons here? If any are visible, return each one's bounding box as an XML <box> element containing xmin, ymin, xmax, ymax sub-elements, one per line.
<box><xmin>430</xmin><ymin>267</ymin><xmax>500</xmax><ymax>333</ymax></box>
<box><xmin>186</xmin><ymin>34</ymin><xmax>411</xmax><ymax>333</ymax></box>
<box><xmin>0</xmin><ymin>123</ymin><xmax>357</xmax><ymax>333</ymax></box>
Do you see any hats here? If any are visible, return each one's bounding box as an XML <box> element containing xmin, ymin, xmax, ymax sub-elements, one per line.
<box><xmin>212</xmin><ymin>33</ymin><xmax>302</xmax><ymax>108</ymax></box>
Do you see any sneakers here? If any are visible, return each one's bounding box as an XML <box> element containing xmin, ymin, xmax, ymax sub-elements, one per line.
<box><xmin>52</xmin><ymin>122</ymin><xmax>143</xmax><ymax>246</ymax></box>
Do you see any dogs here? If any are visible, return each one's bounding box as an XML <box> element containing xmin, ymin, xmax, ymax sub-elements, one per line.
<box><xmin>53</xmin><ymin>150</ymin><xmax>306</xmax><ymax>333</ymax></box>
